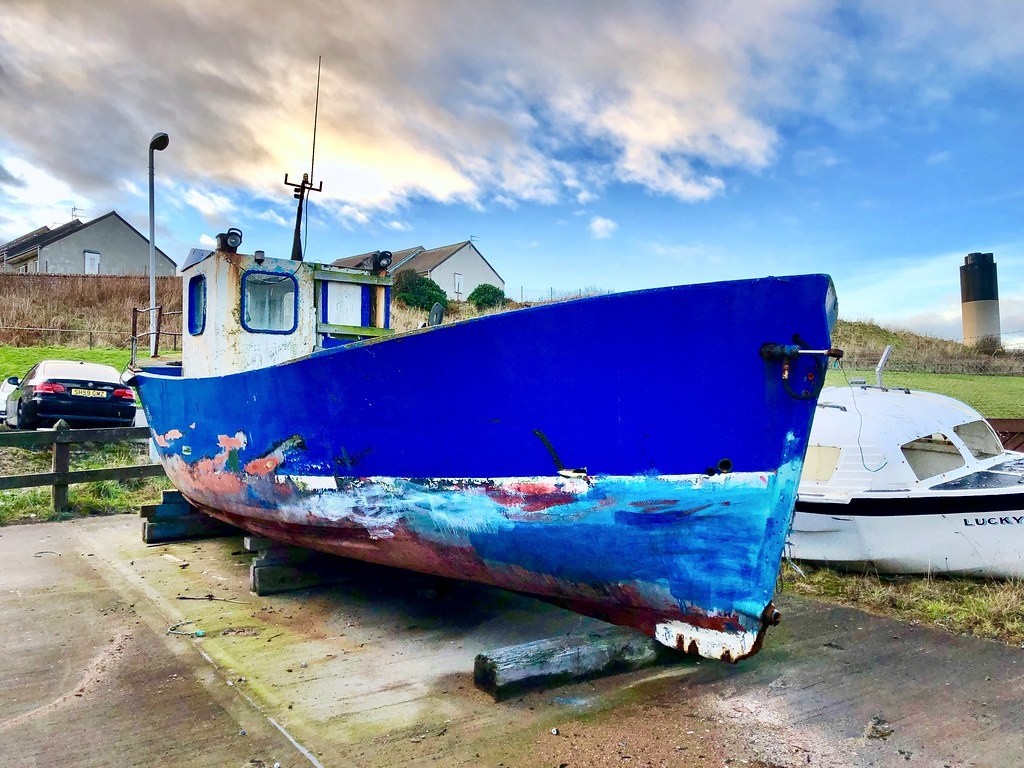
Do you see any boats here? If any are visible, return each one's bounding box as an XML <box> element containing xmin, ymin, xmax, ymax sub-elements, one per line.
<box><xmin>117</xmin><ymin>54</ymin><xmax>849</xmax><ymax>656</ymax></box>
<box><xmin>777</xmin><ymin>342</ymin><xmax>1024</xmax><ymax>583</ymax></box>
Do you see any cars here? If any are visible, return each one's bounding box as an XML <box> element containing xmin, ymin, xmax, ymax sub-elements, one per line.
<box><xmin>0</xmin><ymin>376</ymin><xmax>24</xmax><ymax>424</ymax></box>
<box><xmin>5</xmin><ymin>360</ymin><xmax>138</xmax><ymax>429</ymax></box>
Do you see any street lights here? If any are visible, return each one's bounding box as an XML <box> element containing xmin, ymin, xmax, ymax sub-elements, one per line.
<box><xmin>148</xmin><ymin>132</ymin><xmax>170</xmax><ymax>359</ymax></box>
